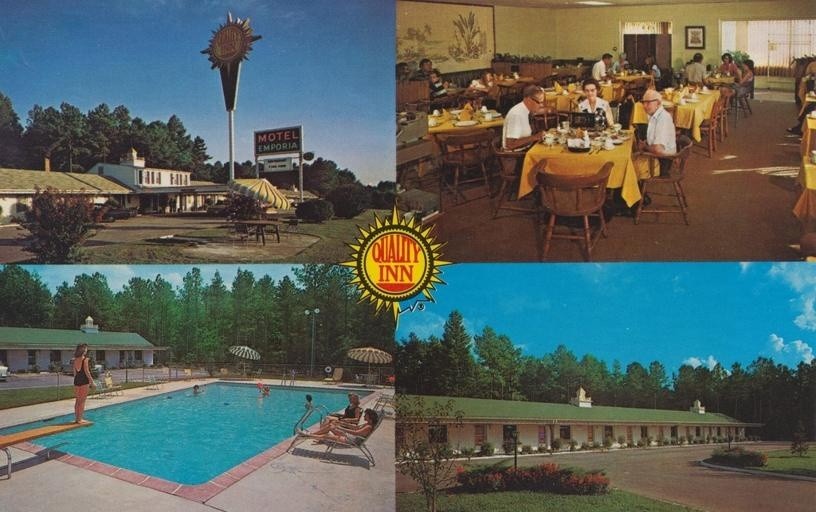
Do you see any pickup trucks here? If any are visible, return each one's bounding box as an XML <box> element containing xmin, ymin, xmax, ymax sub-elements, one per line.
<box><xmin>90</xmin><ymin>203</ymin><xmax>137</xmax><ymax>222</ymax></box>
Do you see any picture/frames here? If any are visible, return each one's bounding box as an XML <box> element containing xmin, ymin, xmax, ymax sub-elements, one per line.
<box><xmin>686</xmin><ymin>25</ymin><xmax>706</xmax><ymax>50</ymax></box>
<box><xmin>397</xmin><ymin>0</ymin><xmax>497</xmax><ymax>83</ymax></box>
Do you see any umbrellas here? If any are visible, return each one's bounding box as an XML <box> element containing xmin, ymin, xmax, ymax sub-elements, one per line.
<box><xmin>347</xmin><ymin>345</ymin><xmax>393</xmax><ymax>382</ymax></box>
<box><xmin>229</xmin><ymin>345</ymin><xmax>261</xmax><ymax>377</ymax></box>
<box><xmin>228</xmin><ymin>175</ymin><xmax>289</xmax><ymax>226</ymax></box>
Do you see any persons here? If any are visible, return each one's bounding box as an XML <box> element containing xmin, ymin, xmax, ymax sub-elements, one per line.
<box><xmin>316</xmin><ymin>393</ymin><xmax>363</xmax><ymax>431</ymax></box>
<box><xmin>71</xmin><ymin>343</ymin><xmax>92</xmax><ymax>424</ymax></box>
<box><xmin>259</xmin><ymin>387</ymin><xmax>269</xmax><ymax>396</ymax></box>
<box><xmin>410</xmin><ymin>49</ymin><xmax>816</xmax><ymax>218</ymax></box>
<box><xmin>305</xmin><ymin>395</ymin><xmax>313</xmax><ymax>412</ymax></box>
<box><xmin>193</xmin><ymin>384</ymin><xmax>199</xmax><ymax>394</ymax></box>
<box><xmin>293</xmin><ymin>408</ymin><xmax>379</xmax><ymax>445</ymax></box>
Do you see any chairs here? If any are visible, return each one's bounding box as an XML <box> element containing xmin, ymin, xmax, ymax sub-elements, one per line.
<box><xmin>233</xmin><ymin>217</ymin><xmax>300</xmax><ymax>248</ymax></box>
<box><xmin>396</xmin><ymin>54</ymin><xmax>755</xmax><ymax>262</ymax></box>
<box><xmin>792</xmin><ymin>56</ymin><xmax>816</xmax><ymax>262</ymax></box>
<box><xmin>286</xmin><ymin>389</ymin><xmax>395</xmax><ymax>464</ymax></box>
<box><xmin>91</xmin><ymin>362</ymin><xmax>395</xmax><ymax>396</ymax></box>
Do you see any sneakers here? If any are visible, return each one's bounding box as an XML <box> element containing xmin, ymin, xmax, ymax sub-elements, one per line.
<box><xmin>785</xmin><ymin>127</ymin><xmax>804</xmax><ymax>135</ymax></box>
<box><xmin>632</xmin><ymin>195</ymin><xmax>652</xmax><ymax>217</ymax></box>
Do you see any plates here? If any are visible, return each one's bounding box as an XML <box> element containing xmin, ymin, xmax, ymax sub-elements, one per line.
<box><xmin>568</xmin><ymin>147</ymin><xmax>591</xmax><ymax>152</ymax></box>
<box><xmin>505</xmin><ymin>79</ymin><xmax>514</xmax><ymax>81</ymax></box>
<box><xmin>591</xmin><ymin>131</ymin><xmax>632</xmax><ymax>150</ymax></box>
<box><xmin>450</xmin><ymin>109</ymin><xmax>502</xmax><ymax>127</ymax></box>
<box><xmin>447</xmin><ymin>89</ymin><xmax>456</xmax><ymax>91</ymax></box>
<box><xmin>699</xmin><ymin>92</ymin><xmax>711</xmax><ymax>94</ymax></box>
<box><xmin>687</xmin><ymin>99</ymin><xmax>699</xmax><ymax>103</ymax></box>
<box><xmin>600</xmin><ymin>84</ymin><xmax>609</xmax><ymax>87</ymax></box>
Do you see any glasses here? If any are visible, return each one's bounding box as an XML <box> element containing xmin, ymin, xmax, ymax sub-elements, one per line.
<box><xmin>529</xmin><ymin>97</ymin><xmax>544</xmax><ymax>104</ymax></box>
<box><xmin>640</xmin><ymin>99</ymin><xmax>659</xmax><ymax>104</ymax></box>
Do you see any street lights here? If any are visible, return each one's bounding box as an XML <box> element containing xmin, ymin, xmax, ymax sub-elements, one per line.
<box><xmin>727</xmin><ymin>431</ymin><xmax>732</xmax><ymax>448</ymax></box>
<box><xmin>510</xmin><ymin>429</ymin><xmax>520</xmax><ymax>470</ymax></box>
<box><xmin>304</xmin><ymin>308</ymin><xmax>319</xmax><ymax>377</ymax></box>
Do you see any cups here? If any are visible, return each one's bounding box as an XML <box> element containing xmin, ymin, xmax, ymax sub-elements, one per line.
<box><xmin>563</xmin><ymin>122</ymin><xmax>569</xmax><ymax>131</ymax></box>
<box><xmin>402</xmin><ymin>102</ymin><xmax>418</xmax><ymax>121</ymax></box>
<box><xmin>432</xmin><ymin>110</ymin><xmax>441</xmax><ymax>117</ymax></box>
<box><xmin>607</xmin><ymin>80</ymin><xmax>612</xmax><ymax>86</ymax></box>
<box><xmin>563</xmin><ymin>90</ymin><xmax>567</xmax><ymax>94</ymax></box>
<box><xmin>681</xmin><ymin>99</ymin><xmax>686</xmax><ymax>105</ymax></box>
<box><xmin>811</xmin><ymin>150</ymin><xmax>816</xmax><ymax>165</ymax></box>
<box><xmin>429</xmin><ymin>120</ymin><xmax>437</xmax><ymax>127</ymax></box>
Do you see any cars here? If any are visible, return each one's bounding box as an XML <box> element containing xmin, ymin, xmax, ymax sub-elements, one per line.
<box><xmin>0</xmin><ymin>365</ymin><xmax>12</xmax><ymax>382</ymax></box>
<box><xmin>61</xmin><ymin>358</ymin><xmax>104</xmax><ymax>375</ymax></box>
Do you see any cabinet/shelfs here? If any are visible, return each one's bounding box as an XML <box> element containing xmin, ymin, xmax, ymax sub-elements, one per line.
<box><xmin>625</xmin><ymin>33</ymin><xmax>673</xmax><ymax>71</ymax></box>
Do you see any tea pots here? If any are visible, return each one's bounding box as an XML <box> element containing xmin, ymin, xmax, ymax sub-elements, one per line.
<box><xmin>594</xmin><ymin>108</ymin><xmax>606</xmax><ymax>130</ymax></box>
